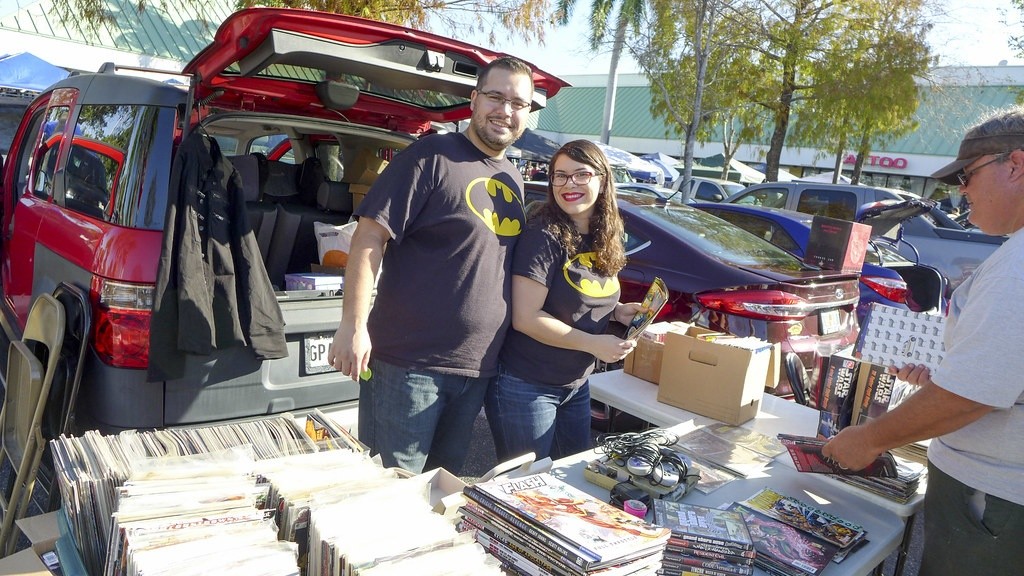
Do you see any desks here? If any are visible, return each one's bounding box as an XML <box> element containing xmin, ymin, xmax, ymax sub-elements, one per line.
<box><xmin>485</xmin><ymin>422</ymin><xmax>906</xmax><ymax>576</ymax></box>
<box><xmin>492</xmin><ymin>367</ymin><xmax>930</xmax><ymax>576</ymax></box>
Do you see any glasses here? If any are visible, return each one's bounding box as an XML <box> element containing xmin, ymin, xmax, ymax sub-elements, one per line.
<box><xmin>477</xmin><ymin>90</ymin><xmax>531</xmax><ymax>109</ymax></box>
<box><xmin>956</xmin><ymin>153</ymin><xmax>1010</xmax><ymax>188</ymax></box>
<box><xmin>551</xmin><ymin>171</ymin><xmax>603</xmax><ymax>188</ymax></box>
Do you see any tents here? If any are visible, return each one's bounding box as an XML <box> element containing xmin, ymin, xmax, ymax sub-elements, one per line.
<box><xmin>1</xmin><ymin>51</ymin><xmax>63</xmax><ymax>96</ymax></box>
<box><xmin>516</xmin><ymin>127</ymin><xmax>866</xmax><ymax>195</ymax></box>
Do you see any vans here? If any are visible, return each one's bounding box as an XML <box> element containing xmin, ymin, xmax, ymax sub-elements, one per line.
<box><xmin>1</xmin><ymin>3</ymin><xmax>573</xmax><ymax>516</ymax></box>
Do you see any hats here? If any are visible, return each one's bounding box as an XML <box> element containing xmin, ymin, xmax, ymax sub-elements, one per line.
<box><xmin>932</xmin><ymin>133</ymin><xmax>1024</xmax><ymax>185</ymax></box>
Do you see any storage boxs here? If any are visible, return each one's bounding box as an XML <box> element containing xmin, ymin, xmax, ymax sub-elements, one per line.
<box><xmin>802</xmin><ymin>214</ymin><xmax>873</xmax><ymax>274</ymax></box>
<box><xmin>657</xmin><ymin>325</ymin><xmax>783</xmax><ymax>427</ymax></box>
<box><xmin>622</xmin><ymin>320</ymin><xmax>697</xmax><ymax>386</ymax></box>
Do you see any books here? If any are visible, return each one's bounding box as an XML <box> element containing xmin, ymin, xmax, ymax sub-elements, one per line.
<box><xmin>621</xmin><ymin>272</ymin><xmax>668</xmax><ymax>345</ymax></box>
<box><xmin>44</xmin><ymin>354</ymin><xmax>900</xmax><ymax>574</ymax></box>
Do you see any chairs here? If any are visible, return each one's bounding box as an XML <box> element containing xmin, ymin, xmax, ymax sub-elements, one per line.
<box><xmin>3</xmin><ymin>280</ymin><xmax>95</xmax><ymax>552</ymax></box>
<box><xmin>266</xmin><ymin>156</ymin><xmax>353</xmax><ymax>288</ymax></box>
<box><xmin>0</xmin><ymin>292</ymin><xmax>67</xmax><ymax>560</ymax></box>
<box><xmin>222</xmin><ymin>152</ymin><xmax>279</xmax><ymax>268</ymax></box>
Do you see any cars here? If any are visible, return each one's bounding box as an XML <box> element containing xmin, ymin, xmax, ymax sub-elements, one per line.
<box><xmin>671</xmin><ymin>201</ymin><xmax>951</xmax><ymax>369</ymax></box>
<box><xmin>681</xmin><ymin>202</ymin><xmax>954</xmax><ymax>366</ymax></box>
<box><xmin>505</xmin><ymin>180</ymin><xmax>863</xmax><ymax>397</ymax></box>
<box><xmin>612</xmin><ymin>179</ymin><xmax>698</xmax><ymax>220</ymax></box>
<box><xmin>954</xmin><ymin>207</ymin><xmax>992</xmax><ymax>234</ymax></box>
<box><xmin>671</xmin><ymin>174</ymin><xmax>763</xmax><ymax>212</ymax></box>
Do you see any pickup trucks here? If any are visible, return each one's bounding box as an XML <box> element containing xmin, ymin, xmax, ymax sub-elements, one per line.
<box><xmin>717</xmin><ymin>177</ymin><xmax>1010</xmax><ymax>297</ymax></box>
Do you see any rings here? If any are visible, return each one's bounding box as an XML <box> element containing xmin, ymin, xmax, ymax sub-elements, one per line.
<box><xmin>838</xmin><ymin>463</ymin><xmax>849</xmax><ymax>470</ymax></box>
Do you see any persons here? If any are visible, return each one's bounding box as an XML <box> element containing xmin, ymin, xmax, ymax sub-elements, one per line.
<box><xmin>816</xmin><ymin>110</ymin><xmax>1024</xmax><ymax>575</ymax></box>
<box><xmin>326</xmin><ymin>55</ymin><xmax>533</xmax><ymax>474</ymax></box>
<box><xmin>486</xmin><ymin>136</ymin><xmax>653</xmax><ymax>469</ymax></box>
